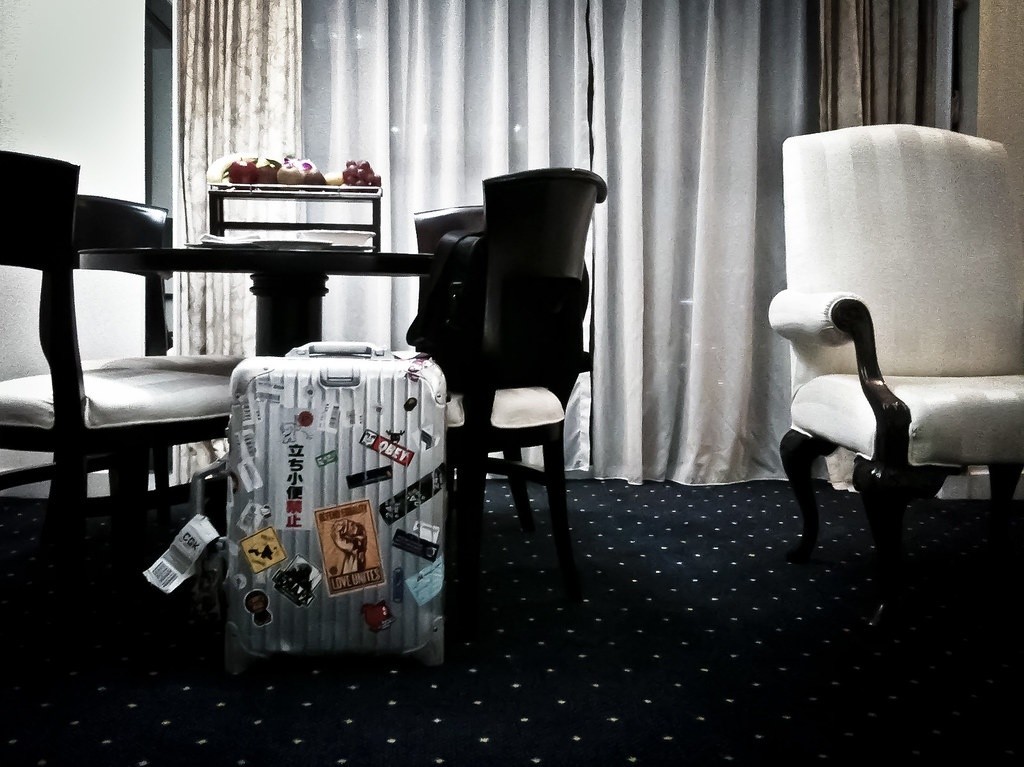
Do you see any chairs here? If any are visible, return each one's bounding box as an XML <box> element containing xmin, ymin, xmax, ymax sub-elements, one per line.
<box><xmin>417</xmin><ymin>168</ymin><xmax>611</xmax><ymax>581</ymax></box>
<box><xmin>0</xmin><ymin>149</ymin><xmax>237</xmax><ymax>622</ymax></box>
<box><xmin>764</xmin><ymin>125</ymin><xmax>1024</xmax><ymax>634</ymax></box>
<box><xmin>66</xmin><ymin>192</ymin><xmax>256</xmax><ymax>578</ymax></box>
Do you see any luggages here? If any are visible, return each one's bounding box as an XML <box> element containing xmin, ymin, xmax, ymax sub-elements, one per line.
<box><xmin>189</xmin><ymin>340</ymin><xmax>446</xmax><ymax>676</ymax></box>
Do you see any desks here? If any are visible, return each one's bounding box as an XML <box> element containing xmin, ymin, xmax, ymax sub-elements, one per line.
<box><xmin>78</xmin><ymin>245</ymin><xmax>436</xmax><ymax>357</ymax></box>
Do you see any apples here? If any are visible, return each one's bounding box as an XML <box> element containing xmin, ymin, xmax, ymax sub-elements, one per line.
<box><xmin>277</xmin><ymin>165</ymin><xmax>301</xmax><ymax>191</ymax></box>
<box><xmin>257</xmin><ymin>166</ymin><xmax>277</xmax><ymax>190</ymax></box>
<box><xmin>229</xmin><ymin>161</ymin><xmax>259</xmax><ymax>190</ymax></box>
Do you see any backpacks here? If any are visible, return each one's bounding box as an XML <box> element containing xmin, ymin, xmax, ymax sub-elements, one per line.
<box><xmin>405</xmin><ymin>229</ymin><xmax>485</xmax><ymax>395</ymax></box>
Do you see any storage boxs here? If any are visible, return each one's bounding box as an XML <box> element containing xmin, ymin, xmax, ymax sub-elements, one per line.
<box><xmin>206</xmin><ymin>181</ymin><xmax>382</xmax><ymax>251</ymax></box>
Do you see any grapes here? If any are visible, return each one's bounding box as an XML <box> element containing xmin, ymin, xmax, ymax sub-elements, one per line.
<box><xmin>343</xmin><ymin>160</ymin><xmax>381</xmax><ymax>186</ymax></box>
